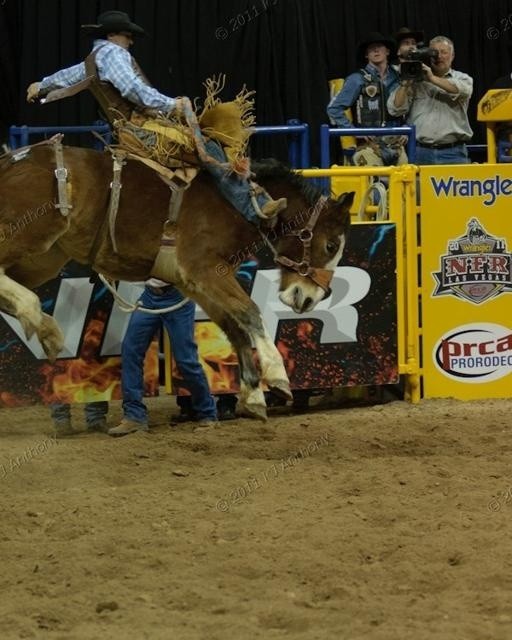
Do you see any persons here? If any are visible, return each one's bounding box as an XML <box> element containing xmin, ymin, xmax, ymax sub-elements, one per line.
<box><xmin>28</xmin><ymin>13</ymin><xmax>510</xmax><ymax>438</ymax></box>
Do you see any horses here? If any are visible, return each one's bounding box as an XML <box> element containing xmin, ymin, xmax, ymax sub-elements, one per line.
<box><xmin>1</xmin><ymin>140</ymin><xmax>356</xmax><ymax>423</ymax></box>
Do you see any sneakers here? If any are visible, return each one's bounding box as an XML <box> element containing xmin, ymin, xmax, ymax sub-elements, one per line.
<box><xmin>54</xmin><ymin>417</ymin><xmax>75</xmax><ymax>435</ymax></box>
<box><xmin>106</xmin><ymin>417</ymin><xmax>148</xmax><ymax>436</ymax></box>
<box><xmin>216</xmin><ymin>406</ymin><xmax>235</xmax><ymax>420</ymax></box>
<box><xmin>192</xmin><ymin>417</ymin><xmax>221</xmax><ymax>432</ymax></box>
<box><xmin>86</xmin><ymin>418</ymin><xmax>108</xmax><ymax>436</ymax></box>
<box><xmin>170</xmin><ymin>407</ymin><xmax>198</xmax><ymax>423</ymax></box>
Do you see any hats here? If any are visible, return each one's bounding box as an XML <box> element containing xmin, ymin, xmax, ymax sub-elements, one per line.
<box><xmin>392</xmin><ymin>27</ymin><xmax>426</xmax><ymax>43</ymax></box>
<box><xmin>357</xmin><ymin>29</ymin><xmax>397</xmax><ymax>56</ymax></box>
<box><xmin>81</xmin><ymin>10</ymin><xmax>144</xmax><ymax>39</ymax></box>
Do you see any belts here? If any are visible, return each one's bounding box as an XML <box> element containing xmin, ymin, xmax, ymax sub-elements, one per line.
<box><xmin>417</xmin><ymin>140</ymin><xmax>460</xmax><ymax>150</ymax></box>
<box><xmin>145</xmin><ymin>284</ymin><xmax>176</xmax><ymax>295</ymax></box>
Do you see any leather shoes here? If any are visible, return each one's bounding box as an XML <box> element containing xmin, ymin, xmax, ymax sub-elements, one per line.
<box><xmin>261</xmin><ymin>196</ymin><xmax>287</xmax><ymax>230</ymax></box>
